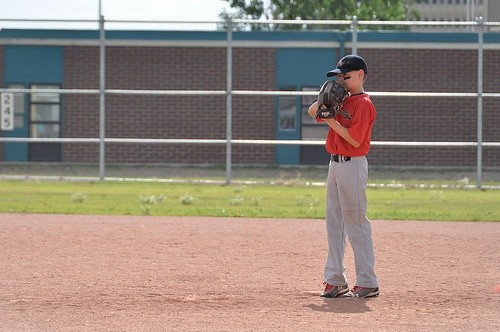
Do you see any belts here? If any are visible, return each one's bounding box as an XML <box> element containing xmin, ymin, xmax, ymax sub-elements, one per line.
<box><xmin>330</xmin><ymin>154</ymin><xmax>352</xmax><ymax>163</ymax></box>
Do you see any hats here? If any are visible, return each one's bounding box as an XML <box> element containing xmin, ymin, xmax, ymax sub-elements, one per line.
<box><xmin>327</xmin><ymin>54</ymin><xmax>368</xmax><ymax>77</ymax></box>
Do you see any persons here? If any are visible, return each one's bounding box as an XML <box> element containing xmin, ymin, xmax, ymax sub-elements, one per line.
<box><xmin>308</xmin><ymin>55</ymin><xmax>380</xmax><ymax>298</ymax></box>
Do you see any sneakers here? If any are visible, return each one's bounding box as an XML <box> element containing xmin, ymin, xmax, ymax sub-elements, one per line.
<box><xmin>320</xmin><ymin>282</ymin><xmax>350</xmax><ymax>298</ymax></box>
<box><xmin>343</xmin><ymin>286</ymin><xmax>380</xmax><ymax>299</ymax></box>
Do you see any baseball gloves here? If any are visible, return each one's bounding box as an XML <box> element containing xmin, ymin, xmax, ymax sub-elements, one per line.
<box><xmin>316</xmin><ymin>80</ymin><xmax>347</xmax><ymax>122</ymax></box>
